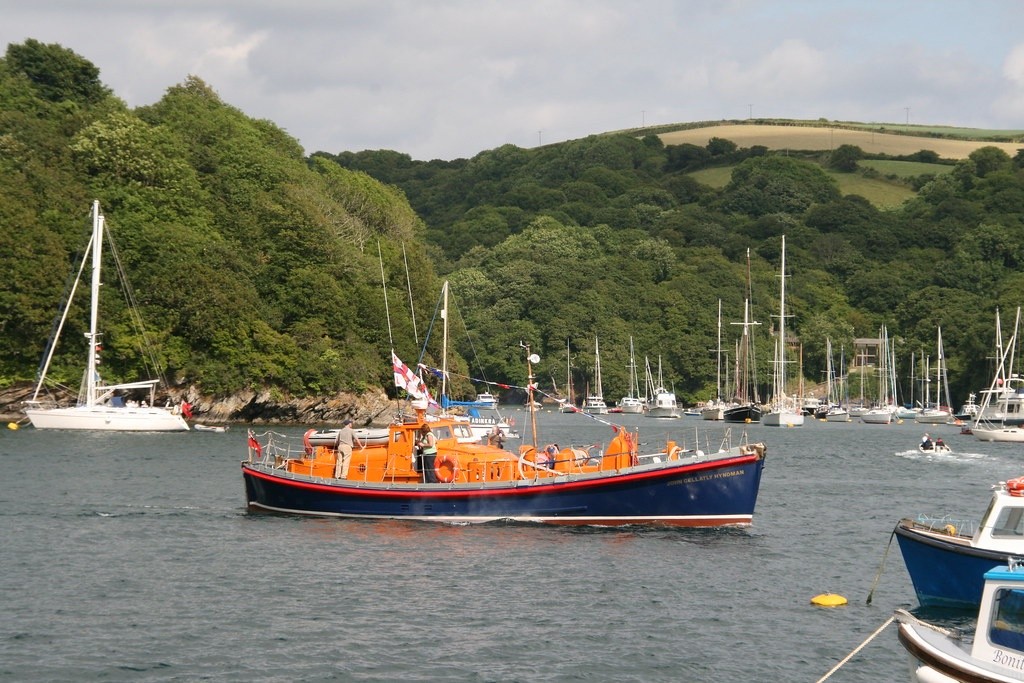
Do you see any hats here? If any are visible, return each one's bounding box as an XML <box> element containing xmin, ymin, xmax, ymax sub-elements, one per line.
<box><xmin>928</xmin><ymin>436</ymin><xmax>932</xmax><ymax>439</ymax></box>
<box><xmin>343</xmin><ymin>420</ymin><xmax>352</xmax><ymax>425</ymax></box>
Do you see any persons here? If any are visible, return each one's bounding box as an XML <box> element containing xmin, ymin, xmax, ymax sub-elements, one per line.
<box><xmin>936</xmin><ymin>438</ymin><xmax>945</xmax><ymax>450</ymax></box>
<box><xmin>277</xmin><ymin>460</ymin><xmax>287</xmax><ymax>470</ymax></box>
<box><xmin>921</xmin><ymin>436</ymin><xmax>933</xmax><ymax>450</ymax></box>
<box><xmin>487</xmin><ymin>426</ymin><xmax>507</xmax><ymax>449</ymax></box>
<box><xmin>543</xmin><ymin>441</ymin><xmax>559</xmax><ymax>470</ymax></box>
<box><xmin>417</xmin><ymin>424</ymin><xmax>439</xmax><ymax>483</ymax></box>
<box><xmin>304</xmin><ymin>448</ymin><xmax>310</xmax><ymax>458</ymax></box>
<box><xmin>165</xmin><ymin>394</ymin><xmax>188</xmax><ymax>407</ymax></box>
<box><xmin>334</xmin><ymin>420</ymin><xmax>363</xmax><ymax>480</ymax></box>
<box><xmin>141</xmin><ymin>399</ymin><xmax>149</xmax><ymax>408</ymax></box>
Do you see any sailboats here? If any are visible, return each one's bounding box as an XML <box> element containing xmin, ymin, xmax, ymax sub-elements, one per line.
<box><xmin>560</xmin><ymin>337</ymin><xmax>577</xmax><ymax>413</ymax></box>
<box><xmin>724</xmin><ymin>234</ymin><xmax>955</xmax><ymax>427</ymax></box>
<box><xmin>970</xmin><ymin>307</ymin><xmax>1024</xmax><ymax>441</ymax></box>
<box><xmin>20</xmin><ymin>199</ymin><xmax>192</xmax><ymax>432</ymax></box>
<box><xmin>700</xmin><ymin>299</ymin><xmax>733</xmax><ymax>420</ymax></box>
<box><xmin>583</xmin><ymin>335</ymin><xmax>682</xmax><ymax>418</ymax></box>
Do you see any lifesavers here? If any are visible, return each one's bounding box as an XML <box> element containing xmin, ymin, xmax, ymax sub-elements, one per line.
<box><xmin>1006</xmin><ymin>476</ymin><xmax>1024</xmax><ymax>496</ymax></box>
<box><xmin>434</xmin><ymin>455</ymin><xmax>459</xmax><ymax>483</ymax></box>
<box><xmin>303</xmin><ymin>429</ymin><xmax>316</xmax><ymax>455</ymax></box>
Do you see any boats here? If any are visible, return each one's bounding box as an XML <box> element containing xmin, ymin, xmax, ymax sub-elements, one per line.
<box><xmin>893</xmin><ymin>556</ymin><xmax>1024</xmax><ymax>683</ymax></box>
<box><xmin>895</xmin><ymin>476</ymin><xmax>1023</xmax><ymax>610</ymax></box>
<box><xmin>918</xmin><ymin>441</ymin><xmax>951</xmax><ymax>456</ymax></box>
<box><xmin>240</xmin><ymin>240</ymin><xmax>767</xmax><ymax>528</ymax></box>
<box><xmin>524</xmin><ymin>401</ymin><xmax>543</xmax><ymax>412</ymax></box>
<box><xmin>946</xmin><ymin>420</ymin><xmax>968</xmax><ymax>427</ymax></box>
<box><xmin>955</xmin><ymin>391</ymin><xmax>979</xmax><ymax>420</ymax></box>
<box><xmin>684</xmin><ymin>412</ymin><xmax>701</xmax><ymax>416</ymax></box>
<box><xmin>304</xmin><ymin>428</ymin><xmax>402</xmax><ymax>448</ymax></box>
<box><xmin>472</xmin><ymin>392</ymin><xmax>498</xmax><ymax>410</ymax></box>
<box><xmin>195</xmin><ymin>424</ymin><xmax>225</xmax><ymax>434</ymax></box>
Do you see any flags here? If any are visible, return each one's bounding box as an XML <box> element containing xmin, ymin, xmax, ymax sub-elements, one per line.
<box><xmin>392</xmin><ymin>350</ymin><xmax>440</xmax><ymax>409</ymax></box>
<box><xmin>248</xmin><ymin>428</ymin><xmax>260</xmax><ymax>457</ymax></box>
